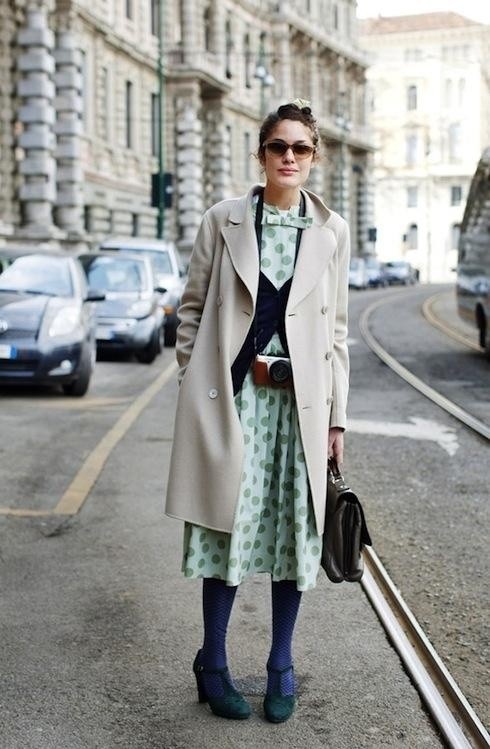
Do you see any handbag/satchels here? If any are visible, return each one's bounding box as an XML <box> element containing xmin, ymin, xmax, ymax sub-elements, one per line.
<box><xmin>321</xmin><ymin>458</ymin><xmax>371</xmax><ymax>582</ymax></box>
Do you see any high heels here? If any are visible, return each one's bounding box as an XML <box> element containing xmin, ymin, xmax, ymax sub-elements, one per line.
<box><xmin>193</xmin><ymin>649</ymin><xmax>250</xmax><ymax>720</ymax></box>
<box><xmin>264</xmin><ymin>664</ymin><xmax>294</xmax><ymax>723</ymax></box>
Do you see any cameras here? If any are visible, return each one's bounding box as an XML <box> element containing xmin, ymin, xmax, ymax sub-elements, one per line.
<box><xmin>253</xmin><ymin>355</ymin><xmax>294</xmax><ymax>388</ymax></box>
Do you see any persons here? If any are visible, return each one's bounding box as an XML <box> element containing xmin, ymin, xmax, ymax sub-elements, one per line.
<box><xmin>164</xmin><ymin>101</ymin><xmax>354</xmax><ymax>727</ymax></box>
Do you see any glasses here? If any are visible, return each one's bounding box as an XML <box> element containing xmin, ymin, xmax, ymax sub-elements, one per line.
<box><xmin>264</xmin><ymin>142</ymin><xmax>314</xmax><ymax>158</ymax></box>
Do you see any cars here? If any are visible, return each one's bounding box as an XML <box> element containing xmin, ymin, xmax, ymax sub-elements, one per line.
<box><xmin>0</xmin><ymin>246</ymin><xmax>106</xmax><ymax>398</ymax></box>
<box><xmin>76</xmin><ymin>237</ymin><xmax>190</xmax><ymax>364</ymax></box>
<box><xmin>348</xmin><ymin>256</ymin><xmax>419</xmax><ymax>291</ymax></box>
<box><xmin>455</xmin><ymin>144</ymin><xmax>490</xmax><ymax>354</ymax></box>
